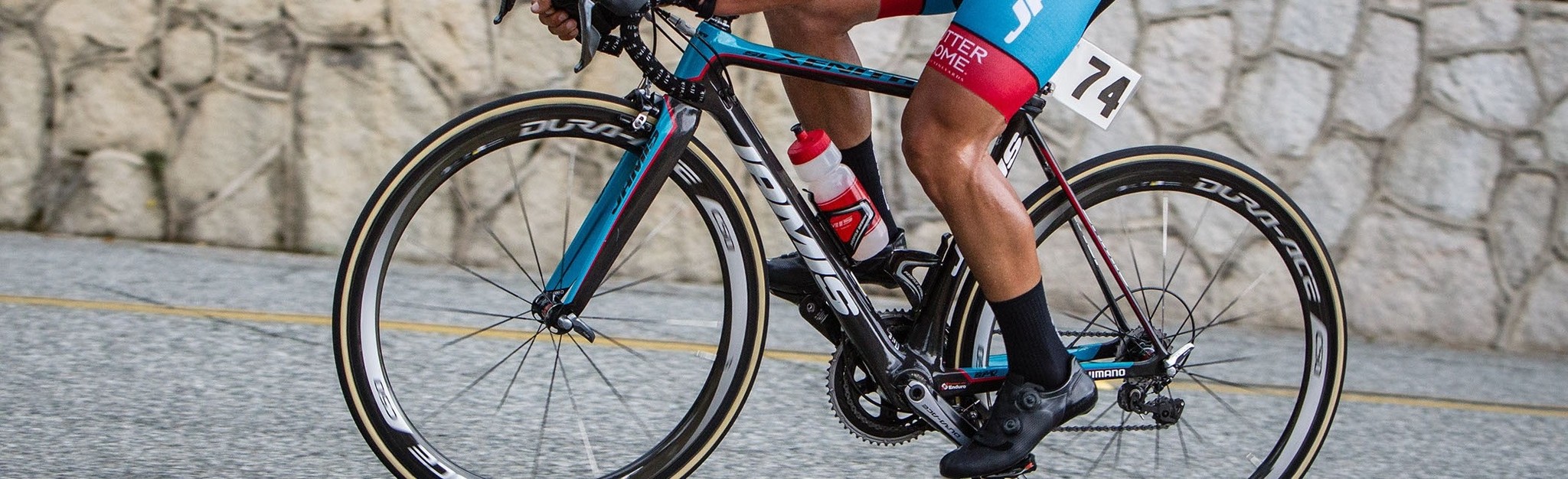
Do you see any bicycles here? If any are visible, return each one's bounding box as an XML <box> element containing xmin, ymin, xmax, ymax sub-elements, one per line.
<box><xmin>332</xmin><ymin>0</ymin><xmax>1349</xmax><ymax>479</ymax></box>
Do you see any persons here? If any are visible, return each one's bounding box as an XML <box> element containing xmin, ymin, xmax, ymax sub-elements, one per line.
<box><xmin>531</xmin><ymin>0</ymin><xmax>1119</xmax><ymax>479</ymax></box>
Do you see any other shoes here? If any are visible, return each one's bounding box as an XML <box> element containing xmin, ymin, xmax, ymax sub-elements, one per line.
<box><xmin>940</xmin><ymin>357</ymin><xmax>1098</xmax><ymax>477</ymax></box>
<box><xmin>765</xmin><ymin>228</ymin><xmax>911</xmax><ymax>294</ymax></box>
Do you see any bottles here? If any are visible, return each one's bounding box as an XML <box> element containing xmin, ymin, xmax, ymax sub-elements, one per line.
<box><xmin>787</xmin><ymin>122</ymin><xmax>889</xmax><ymax>261</ymax></box>
